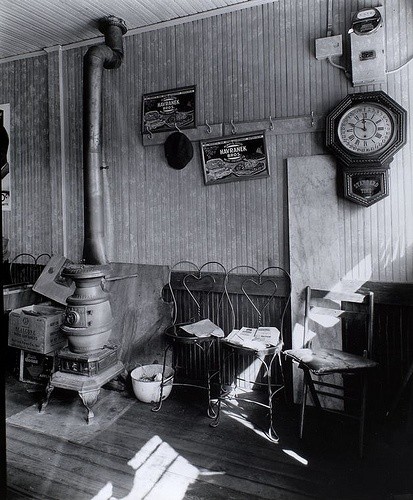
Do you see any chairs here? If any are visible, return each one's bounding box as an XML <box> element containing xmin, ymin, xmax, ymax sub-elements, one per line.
<box><xmin>294</xmin><ymin>286</ymin><xmax>378</xmax><ymax>452</ymax></box>
<box><xmin>222</xmin><ymin>264</ymin><xmax>292</xmax><ymax>440</ymax></box>
<box><xmin>150</xmin><ymin>260</ymin><xmax>226</xmax><ymax>421</ymax></box>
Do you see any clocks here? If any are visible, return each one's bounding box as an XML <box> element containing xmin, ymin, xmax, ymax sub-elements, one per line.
<box><xmin>325</xmin><ymin>90</ymin><xmax>406</xmax><ymax>205</ymax></box>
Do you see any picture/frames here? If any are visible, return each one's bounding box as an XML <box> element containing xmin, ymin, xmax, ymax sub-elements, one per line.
<box><xmin>198</xmin><ymin>129</ymin><xmax>270</xmax><ymax>185</ymax></box>
<box><xmin>141</xmin><ymin>85</ymin><xmax>197</xmax><ymax>130</ymax></box>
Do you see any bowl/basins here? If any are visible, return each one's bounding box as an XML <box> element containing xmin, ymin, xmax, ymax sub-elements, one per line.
<box><xmin>131</xmin><ymin>364</ymin><xmax>174</xmax><ymax>404</ymax></box>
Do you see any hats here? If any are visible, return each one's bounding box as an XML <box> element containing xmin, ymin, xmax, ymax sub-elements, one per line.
<box><xmin>164</xmin><ymin>132</ymin><xmax>193</xmax><ymax>169</ymax></box>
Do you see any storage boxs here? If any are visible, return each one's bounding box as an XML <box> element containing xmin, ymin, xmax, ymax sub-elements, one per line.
<box><xmin>8</xmin><ymin>302</ymin><xmax>67</xmax><ymax>353</ymax></box>
